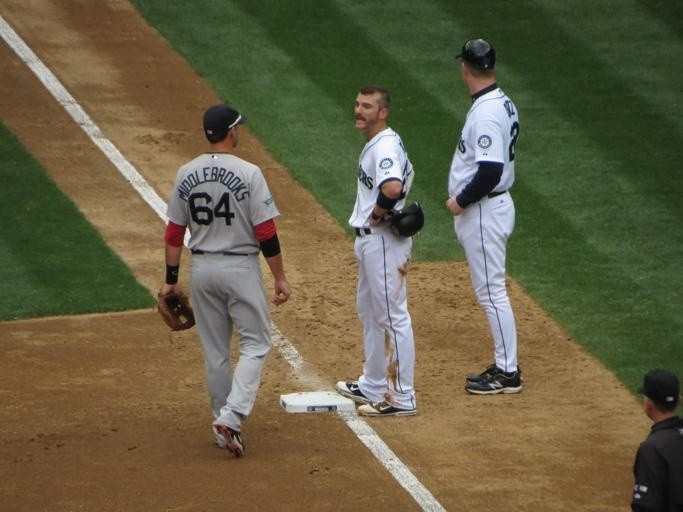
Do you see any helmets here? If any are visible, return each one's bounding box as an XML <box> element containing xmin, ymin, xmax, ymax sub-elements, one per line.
<box><xmin>455</xmin><ymin>37</ymin><xmax>495</xmax><ymax>70</ymax></box>
<box><xmin>385</xmin><ymin>202</ymin><xmax>424</xmax><ymax>238</ymax></box>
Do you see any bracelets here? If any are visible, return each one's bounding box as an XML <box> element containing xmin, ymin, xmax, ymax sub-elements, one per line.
<box><xmin>371</xmin><ymin>210</ymin><xmax>381</xmax><ymax>220</ymax></box>
<box><xmin>165</xmin><ymin>263</ymin><xmax>179</xmax><ymax>285</ymax></box>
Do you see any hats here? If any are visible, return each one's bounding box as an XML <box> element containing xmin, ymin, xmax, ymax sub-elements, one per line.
<box><xmin>203</xmin><ymin>103</ymin><xmax>247</xmax><ymax>135</ymax></box>
<box><xmin>636</xmin><ymin>367</ymin><xmax>679</xmax><ymax>404</ymax></box>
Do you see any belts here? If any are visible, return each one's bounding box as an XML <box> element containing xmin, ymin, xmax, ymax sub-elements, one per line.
<box><xmin>354</xmin><ymin>228</ymin><xmax>370</xmax><ymax>236</ymax></box>
<box><xmin>189</xmin><ymin>248</ymin><xmax>249</xmax><ymax>256</ymax></box>
<box><xmin>489</xmin><ymin>190</ymin><xmax>506</xmax><ymax>198</ymax></box>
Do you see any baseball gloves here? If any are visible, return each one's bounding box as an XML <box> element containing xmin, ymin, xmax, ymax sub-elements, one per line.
<box><xmin>158</xmin><ymin>288</ymin><xmax>195</xmax><ymax>331</ymax></box>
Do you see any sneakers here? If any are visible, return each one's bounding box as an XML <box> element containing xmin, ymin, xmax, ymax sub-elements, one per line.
<box><xmin>213</xmin><ymin>423</ymin><xmax>245</xmax><ymax>458</ymax></box>
<box><xmin>465</xmin><ymin>364</ymin><xmax>524</xmax><ymax>394</ymax></box>
<box><xmin>335</xmin><ymin>380</ymin><xmax>371</xmax><ymax>403</ymax></box>
<box><xmin>357</xmin><ymin>397</ymin><xmax>418</xmax><ymax>418</ymax></box>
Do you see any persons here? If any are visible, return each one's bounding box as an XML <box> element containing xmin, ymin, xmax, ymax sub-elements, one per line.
<box><xmin>629</xmin><ymin>368</ymin><xmax>683</xmax><ymax>512</ymax></box>
<box><xmin>445</xmin><ymin>38</ymin><xmax>523</xmax><ymax>396</ymax></box>
<box><xmin>336</xmin><ymin>87</ymin><xmax>417</xmax><ymax>418</ymax></box>
<box><xmin>157</xmin><ymin>104</ymin><xmax>292</xmax><ymax>458</ymax></box>
